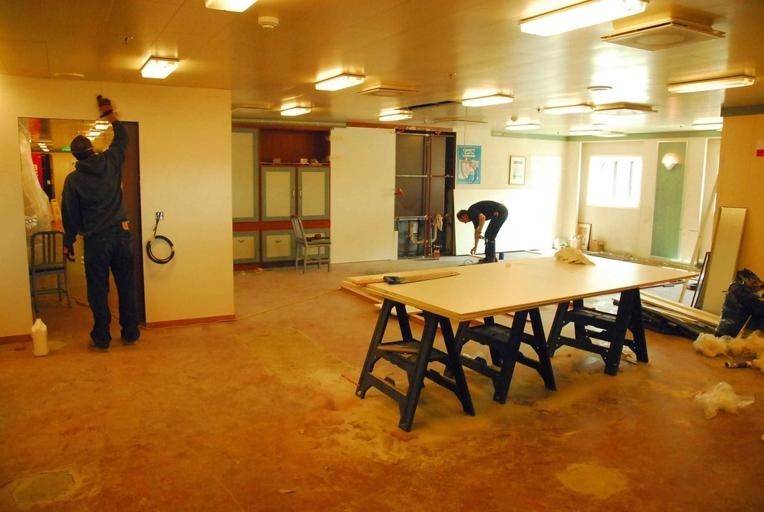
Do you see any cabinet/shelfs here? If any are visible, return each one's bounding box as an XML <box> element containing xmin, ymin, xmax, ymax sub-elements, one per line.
<box><xmin>261</xmin><ymin>166</ymin><xmax>329</xmax><ymax>221</ymax></box>
<box><xmin>233</xmin><ymin>230</ymin><xmax>258</xmax><ymax>263</ymax></box>
<box><xmin>232</xmin><ymin>128</ymin><xmax>260</xmax><ymax>223</ymax></box>
<box><xmin>262</xmin><ymin>229</ymin><xmax>330</xmax><ymax>265</ymax></box>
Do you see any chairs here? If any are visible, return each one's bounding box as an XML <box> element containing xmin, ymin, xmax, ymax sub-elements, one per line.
<box><xmin>289</xmin><ymin>217</ymin><xmax>332</xmax><ymax>273</ymax></box>
<box><xmin>28</xmin><ymin>229</ymin><xmax>74</xmax><ymax>313</ymax></box>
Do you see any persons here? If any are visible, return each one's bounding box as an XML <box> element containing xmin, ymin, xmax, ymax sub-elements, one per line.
<box><xmin>60</xmin><ymin>95</ymin><xmax>140</xmax><ymax>352</ymax></box>
<box><xmin>457</xmin><ymin>200</ymin><xmax>508</xmax><ymax>263</ymax></box>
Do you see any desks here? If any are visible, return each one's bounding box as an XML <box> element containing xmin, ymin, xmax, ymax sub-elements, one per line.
<box><xmin>340</xmin><ymin>252</ymin><xmax>698</xmax><ymax>431</ymax></box>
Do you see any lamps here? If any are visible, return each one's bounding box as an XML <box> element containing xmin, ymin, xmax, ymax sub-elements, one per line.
<box><xmin>204</xmin><ymin>0</ymin><xmax>258</xmax><ymax>14</ymax></box>
<box><xmin>518</xmin><ymin>0</ymin><xmax>649</xmax><ymax>38</ymax></box>
<box><xmin>139</xmin><ymin>56</ymin><xmax>179</xmax><ymax>80</ymax></box>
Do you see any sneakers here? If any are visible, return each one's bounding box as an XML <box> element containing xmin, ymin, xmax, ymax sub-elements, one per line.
<box><xmin>479</xmin><ymin>258</ymin><xmax>497</xmax><ymax>263</ymax></box>
<box><xmin>88</xmin><ymin>337</ymin><xmax>137</xmax><ymax>352</ymax></box>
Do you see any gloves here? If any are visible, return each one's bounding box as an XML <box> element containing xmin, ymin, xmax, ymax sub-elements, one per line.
<box><xmin>97</xmin><ymin>95</ymin><xmax>116</xmax><ymax>123</ymax></box>
<box><xmin>64</xmin><ymin>244</ymin><xmax>75</xmax><ymax>262</ymax></box>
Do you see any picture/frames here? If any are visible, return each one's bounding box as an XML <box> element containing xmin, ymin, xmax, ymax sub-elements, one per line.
<box><xmin>507</xmin><ymin>155</ymin><xmax>526</xmax><ymax>186</ymax></box>
<box><xmin>578</xmin><ymin>221</ymin><xmax>592</xmax><ymax>250</ymax></box>
